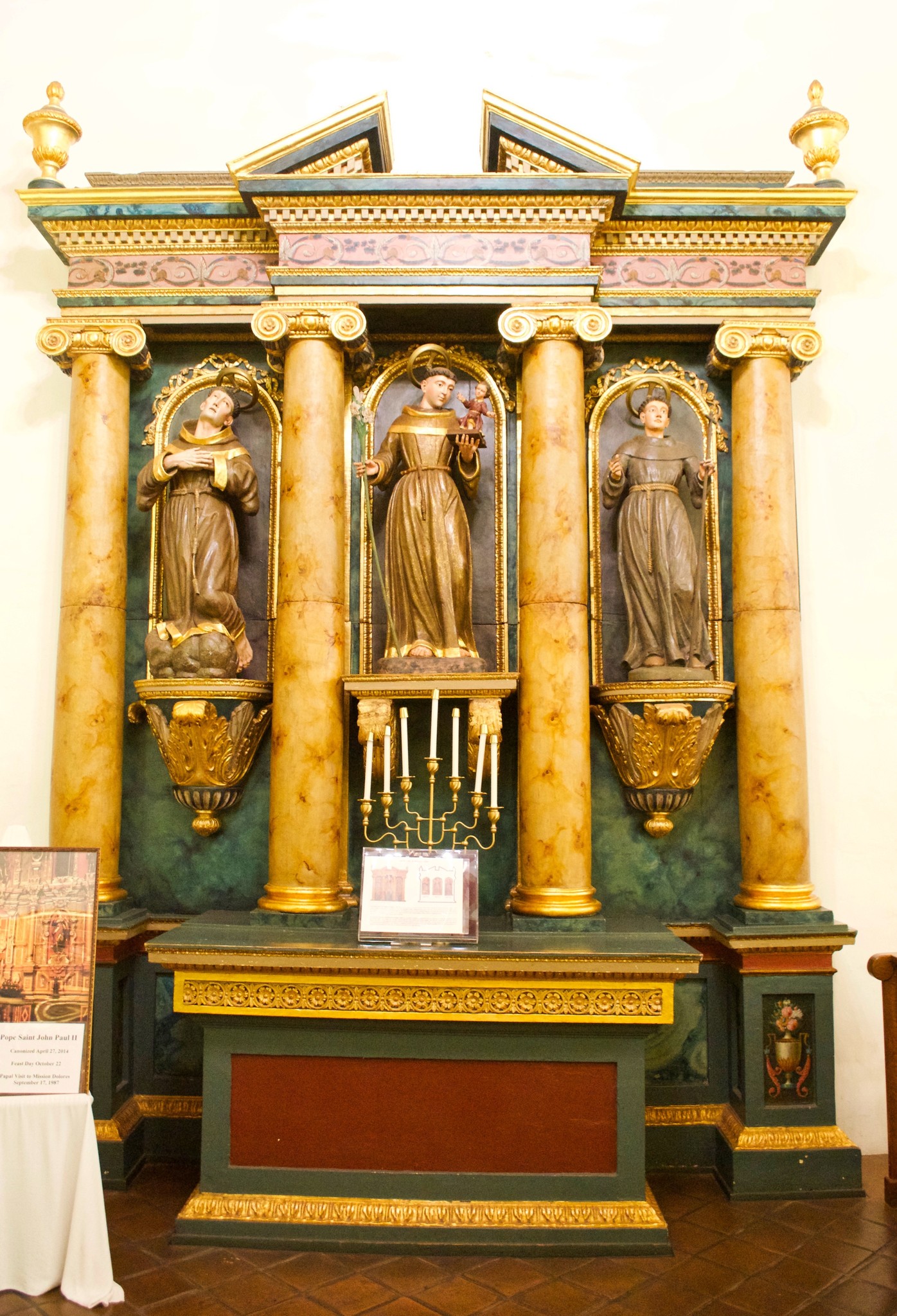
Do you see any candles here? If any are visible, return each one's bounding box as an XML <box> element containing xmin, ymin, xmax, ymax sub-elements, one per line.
<box><xmin>355</xmin><ymin>688</ymin><xmax>505</xmax><ymax>854</ymax></box>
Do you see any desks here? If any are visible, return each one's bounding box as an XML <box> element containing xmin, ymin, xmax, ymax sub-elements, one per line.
<box><xmin>0</xmin><ymin>1093</ymin><xmax>127</xmax><ymax>1309</ymax></box>
<box><xmin>145</xmin><ymin>909</ymin><xmax>706</xmax><ymax>1257</ymax></box>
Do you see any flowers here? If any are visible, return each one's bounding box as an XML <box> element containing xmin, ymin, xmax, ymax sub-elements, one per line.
<box><xmin>350</xmin><ymin>386</ymin><xmax>375</xmax><ymax>425</ymax></box>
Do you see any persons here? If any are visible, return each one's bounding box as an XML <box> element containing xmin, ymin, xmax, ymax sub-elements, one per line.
<box><xmin>136</xmin><ymin>386</ymin><xmax>260</xmax><ymax>672</ymax></box>
<box><xmin>602</xmin><ymin>395</ymin><xmax>717</xmax><ymax>669</ymax></box>
<box><xmin>456</xmin><ymin>381</ymin><xmax>497</xmax><ymax>432</ymax></box>
<box><xmin>353</xmin><ymin>367</ymin><xmax>479</xmax><ymax>658</ymax></box>
